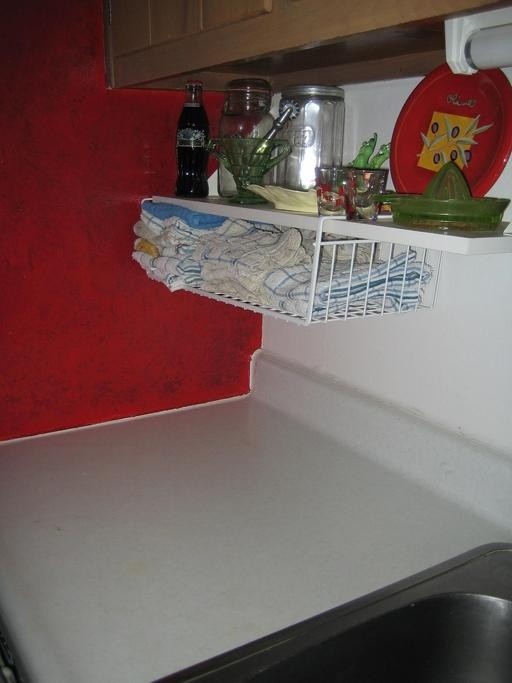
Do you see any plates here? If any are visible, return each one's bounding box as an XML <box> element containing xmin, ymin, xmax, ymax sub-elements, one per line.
<box><xmin>389</xmin><ymin>61</ymin><xmax>512</xmax><ymax>199</ymax></box>
<box><xmin>373</xmin><ymin>194</ymin><xmax>510</xmax><ymax>237</ymax></box>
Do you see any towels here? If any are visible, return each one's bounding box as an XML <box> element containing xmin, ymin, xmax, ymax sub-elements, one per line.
<box><xmin>131</xmin><ymin>252</ymin><xmax>152</xmax><ymax>269</ymax></box>
<box><xmin>134</xmin><ymin>220</ymin><xmax>198</xmax><ymax>247</ymax></box>
<box><xmin>200</xmin><ymin>218</ymin><xmax>319</xmax><ymax>299</ymax></box>
<box><xmin>134</xmin><ymin>237</ymin><xmax>160</xmax><ymax>258</ymax></box>
<box><xmin>247</xmin><ymin>185</ymin><xmax>319</xmax><ymax>212</ymax></box>
<box><xmin>266</xmin><ymin>250</ymin><xmax>432</xmax><ymax>318</ymax></box>
<box><xmin>138</xmin><ymin>209</ymin><xmax>213</xmax><ymax>239</ymax></box>
<box><xmin>144</xmin><ymin>201</ymin><xmax>226</xmax><ymax>228</ymax></box>
<box><xmin>160</xmin><ymin>245</ymin><xmax>195</xmax><ymax>256</ymax></box>
<box><xmin>152</xmin><ymin>270</ymin><xmax>201</xmax><ymax>292</ymax></box>
<box><xmin>153</xmin><ymin>256</ymin><xmax>202</xmax><ymax>276</ymax></box>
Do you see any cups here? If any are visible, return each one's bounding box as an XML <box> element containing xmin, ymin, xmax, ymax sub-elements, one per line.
<box><xmin>346</xmin><ymin>169</ymin><xmax>388</xmax><ymax>221</ymax></box>
<box><xmin>317</xmin><ymin>167</ymin><xmax>348</xmax><ymax>216</ymax></box>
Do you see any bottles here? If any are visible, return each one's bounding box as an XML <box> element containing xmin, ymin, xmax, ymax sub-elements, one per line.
<box><xmin>217</xmin><ymin>78</ymin><xmax>274</xmax><ymax>200</ymax></box>
<box><xmin>278</xmin><ymin>84</ymin><xmax>346</xmax><ymax>193</ymax></box>
<box><xmin>175</xmin><ymin>80</ymin><xmax>210</xmax><ymax>200</ymax></box>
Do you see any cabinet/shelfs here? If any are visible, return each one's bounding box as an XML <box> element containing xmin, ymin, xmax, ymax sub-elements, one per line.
<box><xmin>101</xmin><ymin>0</ymin><xmax>511</xmax><ymax>93</ymax></box>
<box><xmin>155</xmin><ymin>199</ymin><xmax>512</xmax><ymax>257</ymax></box>
